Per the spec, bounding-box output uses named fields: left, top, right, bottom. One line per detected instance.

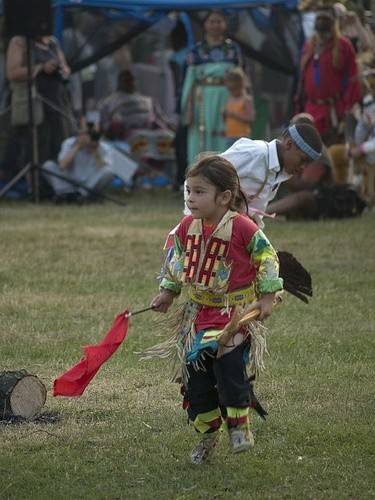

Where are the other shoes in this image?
left=56, top=192, right=106, bottom=206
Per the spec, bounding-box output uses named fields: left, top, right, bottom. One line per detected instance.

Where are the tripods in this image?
left=0, top=36, right=127, bottom=207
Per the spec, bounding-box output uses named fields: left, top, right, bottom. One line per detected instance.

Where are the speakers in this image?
left=3, top=0, right=54, bottom=35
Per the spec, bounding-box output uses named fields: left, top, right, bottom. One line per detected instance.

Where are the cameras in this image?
left=82, top=121, right=101, bottom=141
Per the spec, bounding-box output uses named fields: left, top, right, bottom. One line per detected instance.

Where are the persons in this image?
left=0, top=0, right=374, bottom=219
left=150, top=125, right=323, bottom=465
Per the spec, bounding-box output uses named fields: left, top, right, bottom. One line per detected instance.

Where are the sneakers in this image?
left=192, top=433, right=220, bottom=466
left=231, top=429, right=255, bottom=455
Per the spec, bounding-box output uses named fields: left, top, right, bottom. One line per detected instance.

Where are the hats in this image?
left=313, top=16, right=335, bottom=32
